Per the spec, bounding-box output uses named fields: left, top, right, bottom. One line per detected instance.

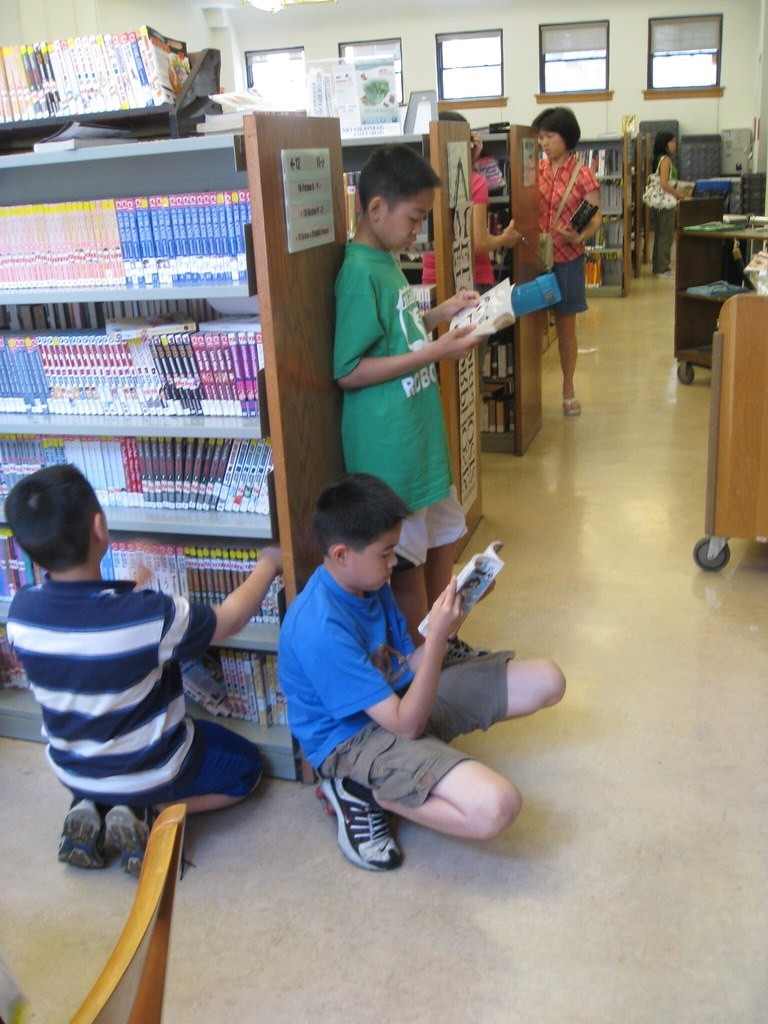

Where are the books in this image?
left=343, top=169, right=436, bottom=342
left=539, top=150, right=635, bottom=288
left=449, top=277, right=515, bottom=338
left=570, top=200, right=599, bottom=234
left=473, top=155, right=516, bottom=433
left=0, top=189, right=287, bottom=730
left=417, top=541, right=504, bottom=639
left=1, top=24, right=307, bottom=155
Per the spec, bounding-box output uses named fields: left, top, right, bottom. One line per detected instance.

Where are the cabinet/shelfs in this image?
left=0, top=48, right=768, bottom=784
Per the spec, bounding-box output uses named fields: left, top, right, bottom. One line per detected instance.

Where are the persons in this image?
left=652, top=131, right=685, bottom=280
left=277, top=473, right=565, bottom=873
left=532, top=106, right=602, bottom=415
left=421, top=111, right=521, bottom=396
left=5, top=463, right=283, bottom=881
left=332, top=144, right=491, bottom=663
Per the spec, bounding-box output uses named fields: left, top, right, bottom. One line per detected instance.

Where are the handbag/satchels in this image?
left=538, top=232, right=554, bottom=273
left=496, top=235, right=562, bottom=318
left=641, top=154, right=679, bottom=210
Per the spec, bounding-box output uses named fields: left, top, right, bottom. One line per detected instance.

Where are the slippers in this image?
left=562, top=397, right=581, bottom=415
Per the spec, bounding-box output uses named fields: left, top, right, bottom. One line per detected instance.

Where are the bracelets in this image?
left=581, top=233, right=585, bottom=242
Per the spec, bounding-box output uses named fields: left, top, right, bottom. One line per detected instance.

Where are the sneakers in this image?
left=441, top=634, right=491, bottom=668
left=314, top=776, right=403, bottom=872
left=56, top=798, right=106, bottom=869
left=103, top=804, right=189, bottom=881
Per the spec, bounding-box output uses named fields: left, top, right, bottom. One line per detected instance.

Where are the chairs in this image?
left=73, top=802, right=188, bottom=1024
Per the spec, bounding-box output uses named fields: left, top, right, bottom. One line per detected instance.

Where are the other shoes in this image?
left=655, top=269, right=675, bottom=280
